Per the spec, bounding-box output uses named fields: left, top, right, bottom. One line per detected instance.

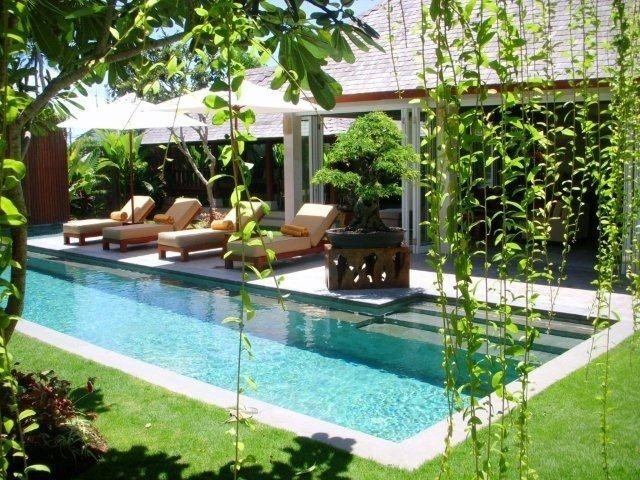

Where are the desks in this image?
left=324, top=244, right=411, bottom=290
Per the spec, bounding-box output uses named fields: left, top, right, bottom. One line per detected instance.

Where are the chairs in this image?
left=522, top=188, right=594, bottom=242
left=225, top=202, right=346, bottom=273
left=63, top=195, right=155, bottom=245
left=158, top=200, right=266, bottom=261
left=103, top=198, right=203, bottom=252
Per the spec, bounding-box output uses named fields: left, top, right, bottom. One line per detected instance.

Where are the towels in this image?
left=211, top=221, right=235, bottom=231
left=281, top=224, right=309, bottom=237
left=153, top=214, right=174, bottom=225
left=110, top=211, right=128, bottom=221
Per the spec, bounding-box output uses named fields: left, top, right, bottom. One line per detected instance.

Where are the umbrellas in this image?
left=56, top=91, right=208, bottom=220
left=156, top=72, right=334, bottom=184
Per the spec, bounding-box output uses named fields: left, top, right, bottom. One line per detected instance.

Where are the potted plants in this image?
left=310, top=111, right=421, bottom=249
